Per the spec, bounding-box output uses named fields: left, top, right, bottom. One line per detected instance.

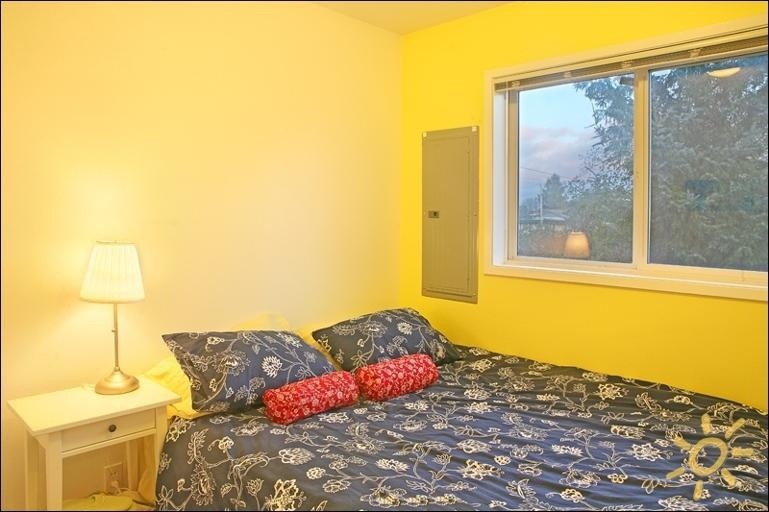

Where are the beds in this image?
left=121, top=324, right=769, bottom=511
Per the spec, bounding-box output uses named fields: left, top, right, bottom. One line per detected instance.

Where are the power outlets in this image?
left=105, top=462, right=123, bottom=494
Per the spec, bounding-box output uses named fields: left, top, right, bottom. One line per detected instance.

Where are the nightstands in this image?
left=6, top=369, right=184, bottom=509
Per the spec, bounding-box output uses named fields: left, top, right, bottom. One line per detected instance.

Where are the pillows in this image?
left=251, top=368, right=362, bottom=429
left=157, top=328, right=338, bottom=417
left=295, top=307, right=392, bottom=374
left=348, top=350, right=442, bottom=401
left=142, top=312, right=337, bottom=422
left=307, top=301, right=464, bottom=377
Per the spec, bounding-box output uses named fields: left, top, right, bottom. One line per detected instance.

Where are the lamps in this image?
left=74, top=236, right=157, bottom=394
